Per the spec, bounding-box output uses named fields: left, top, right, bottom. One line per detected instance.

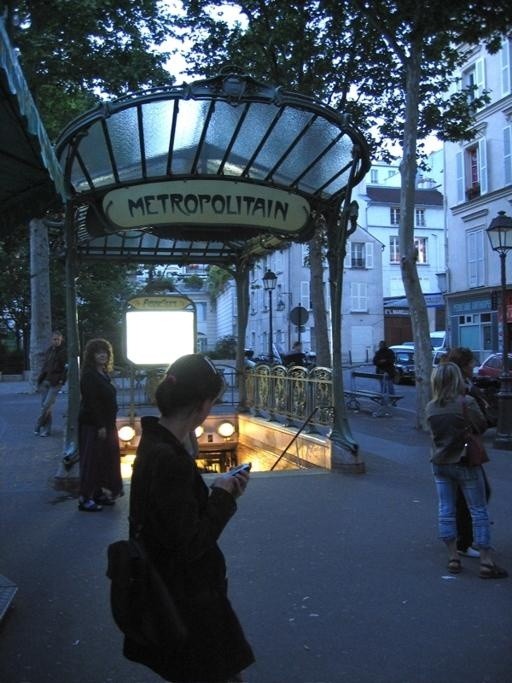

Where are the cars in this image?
left=388, top=328, right=512, bottom=381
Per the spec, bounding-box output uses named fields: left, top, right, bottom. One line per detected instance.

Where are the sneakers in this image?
left=457, top=543, right=481, bottom=559
left=32, top=428, right=48, bottom=437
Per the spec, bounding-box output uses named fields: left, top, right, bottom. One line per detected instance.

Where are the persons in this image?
left=372, top=340, right=397, bottom=406
left=76, top=337, right=126, bottom=512
left=283, top=340, right=306, bottom=367
left=33, top=329, right=68, bottom=438
left=425, top=360, right=508, bottom=580
left=121, top=353, right=257, bottom=682
left=446, top=346, right=492, bottom=559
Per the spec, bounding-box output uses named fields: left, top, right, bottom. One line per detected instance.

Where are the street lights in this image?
left=261, top=268, right=280, bottom=367
left=484, top=209, right=512, bottom=451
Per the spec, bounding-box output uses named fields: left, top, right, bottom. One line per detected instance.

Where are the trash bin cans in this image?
left=471, top=375, right=501, bottom=428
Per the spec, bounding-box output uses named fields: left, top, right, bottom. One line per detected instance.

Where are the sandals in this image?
left=475, top=561, right=506, bottom=580
left=76, top=489, right=115, bottom=511
left=448, top=556, right=464, bottom=574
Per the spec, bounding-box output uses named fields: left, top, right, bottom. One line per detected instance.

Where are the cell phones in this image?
left=222, top=464, right=250, bottom=480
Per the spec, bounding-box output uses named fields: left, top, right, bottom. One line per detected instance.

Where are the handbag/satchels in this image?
left=465, top=429, right=490, bottom=466
left=103, top=540, right=200, bottom=672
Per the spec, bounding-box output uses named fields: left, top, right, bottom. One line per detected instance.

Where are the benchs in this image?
left=342, top=371, right=405, bottom=418
